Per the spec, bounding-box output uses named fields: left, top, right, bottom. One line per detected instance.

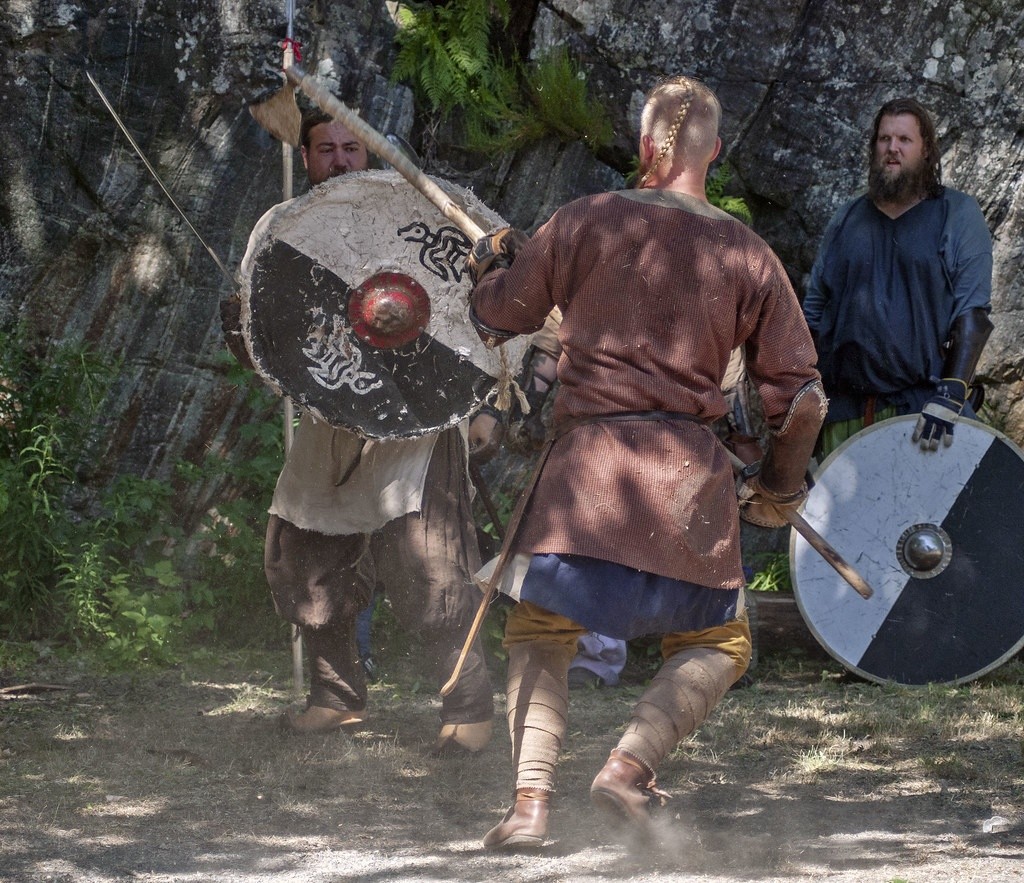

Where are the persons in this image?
left=264, top=82, right=497, bottom=763
left=467, top=75, right=830, bottom=874
left=802, top=97, right=995, bottom=685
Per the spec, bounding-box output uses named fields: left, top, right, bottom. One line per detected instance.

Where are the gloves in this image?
left=508, top=405, right=545, bottom=457
left=468, top=228, right=529, bottom=287
left=912, top=378, right=972, bottom=451
left=219, top=296, right=255, bottom=370
left=737, top=475, right=809, bottom=528
left=468, top=413, right=505, bottom=465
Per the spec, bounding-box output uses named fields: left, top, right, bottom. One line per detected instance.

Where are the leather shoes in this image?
left=590, top=748, right=674, bottom=844
left=483, top=786, right=554, bottom=851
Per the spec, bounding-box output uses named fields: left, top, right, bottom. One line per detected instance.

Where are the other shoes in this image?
left=568, top=668, right=601, bottom=691
left=432, top=719, right=492, bottom=756
left=279, top=705, right=363, bottom=736
left=413, top=671, right=439, bottom=694
